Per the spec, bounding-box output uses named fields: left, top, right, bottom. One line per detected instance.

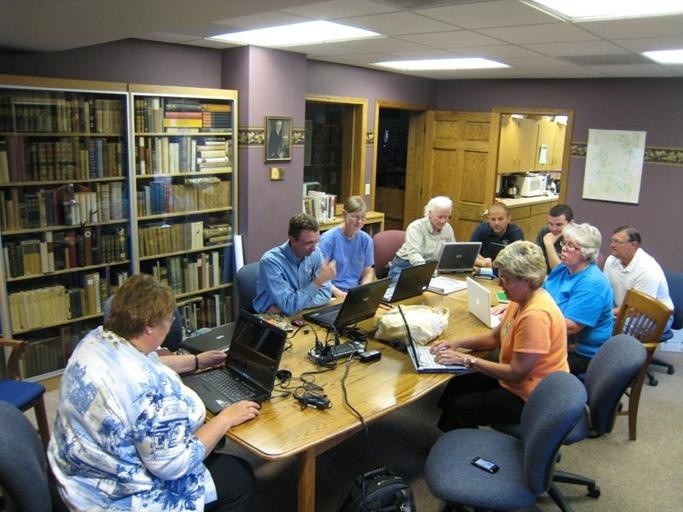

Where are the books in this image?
left=303, top=190, right=338, bottom=223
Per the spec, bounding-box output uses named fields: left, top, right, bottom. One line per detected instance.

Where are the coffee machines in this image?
left=500, top=174, right=519, bottom=199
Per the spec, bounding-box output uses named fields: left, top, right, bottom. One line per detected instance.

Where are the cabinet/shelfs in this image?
left=495, top=115, right=538, bottom=177
left=533, top=119, right=567, bottom=173
left=0, top=72, right=240, bottom=388
left=493, top=198, right=559, bottom=247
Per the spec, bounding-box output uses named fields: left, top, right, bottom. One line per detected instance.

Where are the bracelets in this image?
left=463, top=355, right=476, bottom=368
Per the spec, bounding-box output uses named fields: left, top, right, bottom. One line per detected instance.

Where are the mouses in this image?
left=291, top=319, right=305, bottom=328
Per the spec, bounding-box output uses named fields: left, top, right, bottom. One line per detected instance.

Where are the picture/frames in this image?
left=264, top=114, right=292, bottom=164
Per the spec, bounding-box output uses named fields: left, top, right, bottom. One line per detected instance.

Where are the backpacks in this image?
left=335, top=463, right=417, bottom=511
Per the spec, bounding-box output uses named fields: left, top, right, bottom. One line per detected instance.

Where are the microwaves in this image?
left=514, top=173, right=547, bottom=197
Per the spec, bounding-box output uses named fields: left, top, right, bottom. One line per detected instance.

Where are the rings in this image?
left=443, top=353, right=448, bottom=359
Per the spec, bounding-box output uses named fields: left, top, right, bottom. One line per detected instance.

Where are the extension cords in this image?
left=308, top=339, right=367, bottom=365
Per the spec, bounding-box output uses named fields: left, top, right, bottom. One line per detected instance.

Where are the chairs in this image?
left=487, top=332, right=646, bottom=512
left=643, top=272, right=682, bottom=390
left=373, top=228, right=410, bottom=280
left=0, top=399, right=71, bottom=511
left=0, top=337, right=51, bottom=457
left=610, top=286, right=673, bottom=442
left=422, top=369, right=589, bottom=512
left=102, top=290, right=185, bottom=355
left=234, top=260, right=262, bottom=313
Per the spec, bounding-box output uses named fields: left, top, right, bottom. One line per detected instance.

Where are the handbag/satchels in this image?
left=373, top=303, right=450, bottom=348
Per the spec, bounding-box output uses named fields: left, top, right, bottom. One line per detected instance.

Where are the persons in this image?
left=536, top=205, right=574, bottom=275
left=317, top=196, right=376, bottom=299
left=603, top=226, right=675, bottom=339
left=387, top=196, right=456, bottom=283
left=47, top=274, right=261, bottom=512
left=253, top=213, right=337, bottom=314
left=429, top=240, right=571, bottom=433
left=539, top=222, right=615, bottom=376
left=471, top=202, right=524, bottom=267
left=268, top=119, right=288, bottom=158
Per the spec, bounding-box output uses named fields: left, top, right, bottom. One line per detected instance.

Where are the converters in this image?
left=299, top=392, right=330, bottom=411
left=348, top=332, right=368, bottom=342
left=360, top=349, right=381, bottom=362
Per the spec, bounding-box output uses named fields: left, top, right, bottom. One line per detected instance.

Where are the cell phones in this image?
left=470, top=455, right=500, bottom=474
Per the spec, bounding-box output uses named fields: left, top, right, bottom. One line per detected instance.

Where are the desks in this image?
left=317, top=211, right=386, bottom=239
left=173, top=261, right=525, bottom=511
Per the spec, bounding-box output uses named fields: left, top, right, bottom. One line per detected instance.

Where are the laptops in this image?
left=181, top=322, right=244, bottom=356
left=380, top=260, right=438, bottom=303
left=397, top=305, right=470, bottom=374
left=435, top=242, right=483, bottom=274
left=466, top=276, right=502, bottom=329
left=302, top=275, right=393, bottom=330
left=180, top=307, right=287, bottom=417
